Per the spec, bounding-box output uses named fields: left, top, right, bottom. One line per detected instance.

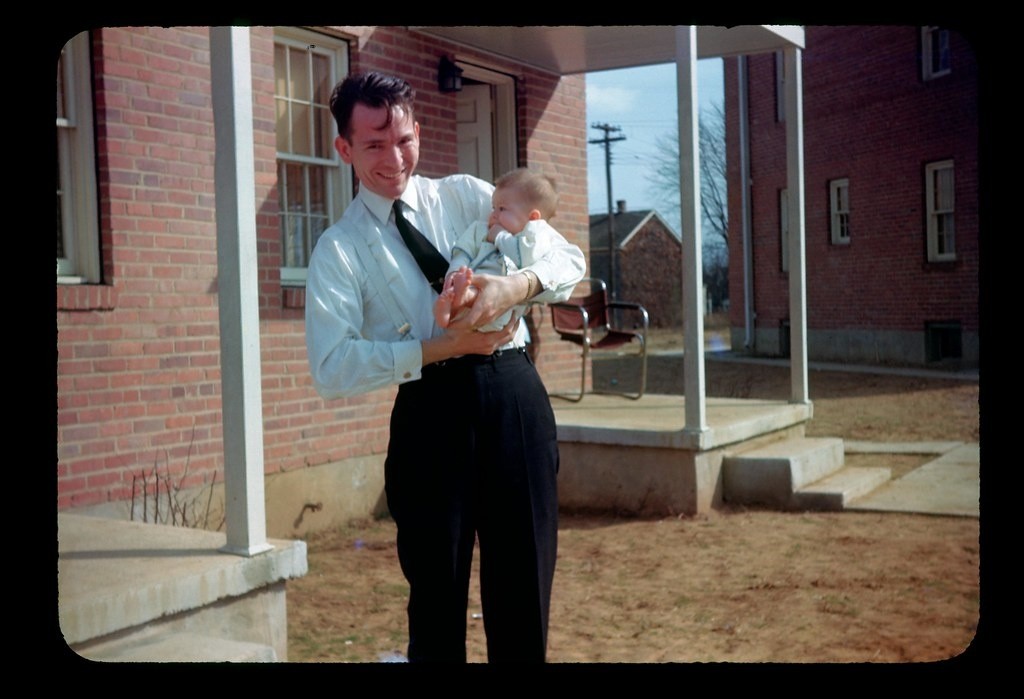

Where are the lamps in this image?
left=438, top=57, right=463, bottom=95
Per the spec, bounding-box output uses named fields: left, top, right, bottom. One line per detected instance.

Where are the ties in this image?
left=391, top=199, right=450, bottom=293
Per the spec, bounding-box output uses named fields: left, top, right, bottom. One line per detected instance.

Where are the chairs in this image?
left=547, top=278, right=649, bottom=403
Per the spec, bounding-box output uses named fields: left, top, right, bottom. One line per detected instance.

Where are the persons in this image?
left=303, top=70, right=587, bottom=663
left=432, top=168, right=559, bottom=331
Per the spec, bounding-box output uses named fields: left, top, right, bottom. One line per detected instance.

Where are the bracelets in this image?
left=522, top=271, right=533, bottom=300
